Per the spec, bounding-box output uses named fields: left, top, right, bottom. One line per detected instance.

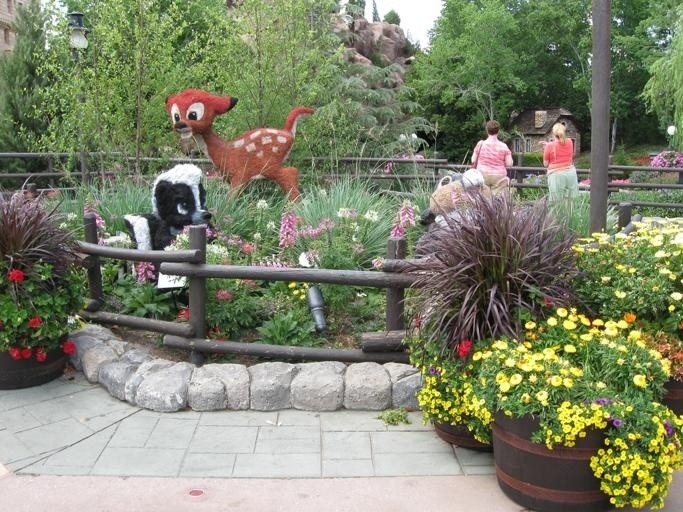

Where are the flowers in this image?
left=654, top=153, right=683, bottom=170
left=402, top=310, right=588, bottom=446
left=621, top=308, right=683, bottom=385
left=471, top=308, right=680, bottom=508
left=0, top=266, right=87, bottom=362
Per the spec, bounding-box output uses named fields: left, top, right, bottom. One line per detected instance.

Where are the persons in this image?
left=538, top=122, right=579, bottom=202
left=471, top=120, right=513, bottom=184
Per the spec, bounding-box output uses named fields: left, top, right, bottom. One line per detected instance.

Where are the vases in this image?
left=1, top=345, right=71, bottom=391
left=485, top=395, right=618, bottom=508
left=652, top=374, right=683, bottom=418
left=658, top=168, right=677, bottom=187
left=422, top=385, right=494, bottom=453
